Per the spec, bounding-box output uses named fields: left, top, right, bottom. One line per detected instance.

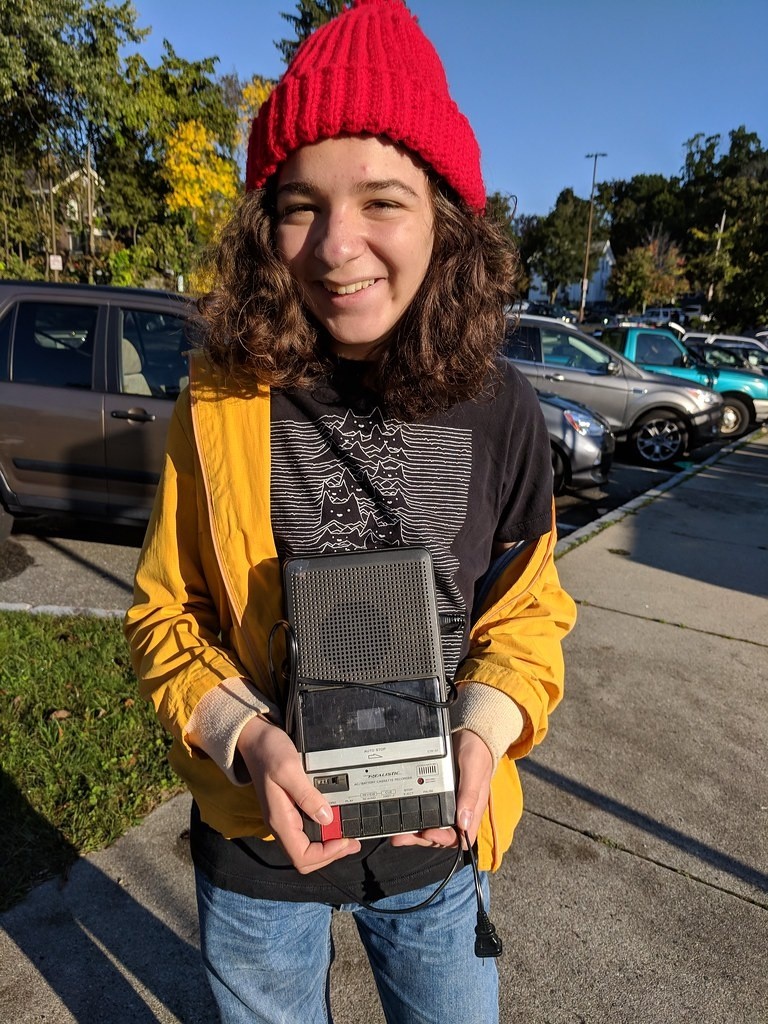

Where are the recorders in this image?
left=284, top=544, right=460, bottom=842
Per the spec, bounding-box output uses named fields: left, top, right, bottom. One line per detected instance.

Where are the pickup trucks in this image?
left=544, top=320, right=768, bottom=441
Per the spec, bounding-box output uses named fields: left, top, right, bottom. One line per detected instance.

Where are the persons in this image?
left=120, top=0, right=580, bottom=1024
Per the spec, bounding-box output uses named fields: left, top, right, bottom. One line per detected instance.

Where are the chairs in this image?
left=121, top=336, right=153, bottom=396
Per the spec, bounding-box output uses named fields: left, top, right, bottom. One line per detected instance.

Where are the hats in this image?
left=244, top=1, right=486, bottom=221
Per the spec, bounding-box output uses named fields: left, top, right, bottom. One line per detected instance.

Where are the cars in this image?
left=501, top=299, right=530, bottom=314
left=534, top=387, right=618, bottom=498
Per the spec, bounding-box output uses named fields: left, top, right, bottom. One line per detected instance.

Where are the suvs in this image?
left=0, top=278, right=207, bottom=540
left=496, top=309, right=727, bottom=470
left=527, top=300, right=579, bottom=326
left=628, top=306, right=690, bottom=329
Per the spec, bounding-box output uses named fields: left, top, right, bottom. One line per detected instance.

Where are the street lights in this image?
left=578, top=152, right=609, bottom=324
